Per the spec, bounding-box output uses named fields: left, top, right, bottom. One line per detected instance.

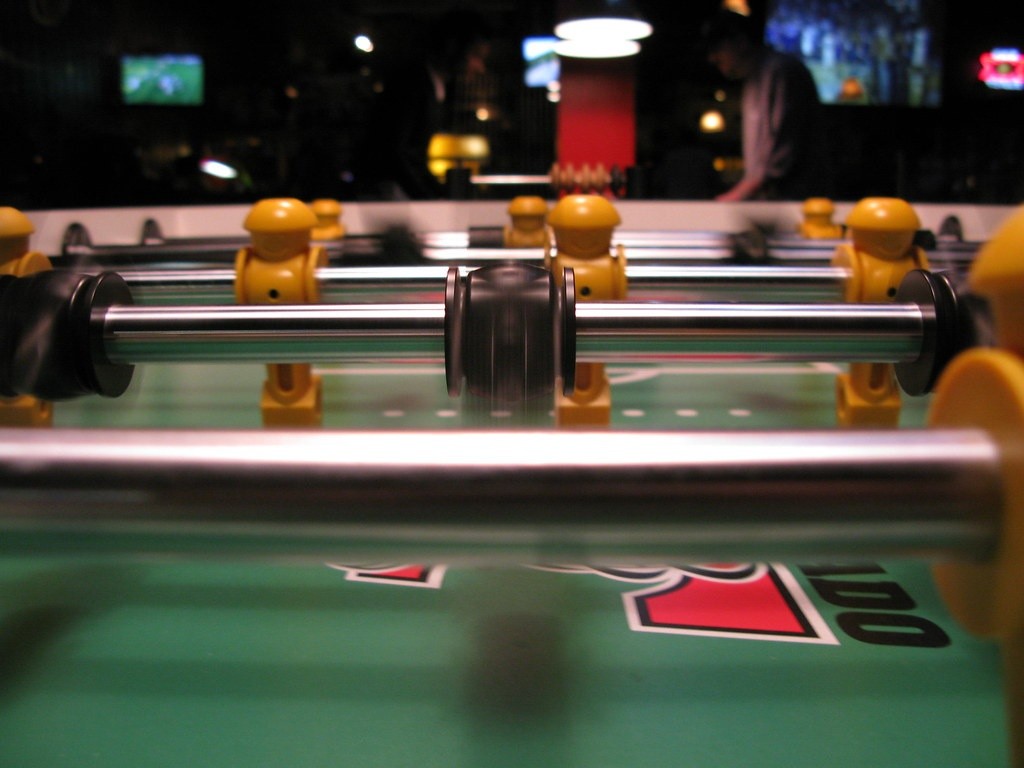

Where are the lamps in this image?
left=551, top=0, right=652, bottom=59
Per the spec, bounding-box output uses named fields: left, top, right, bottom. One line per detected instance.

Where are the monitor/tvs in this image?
left=120, top=55, right=203, bottom=104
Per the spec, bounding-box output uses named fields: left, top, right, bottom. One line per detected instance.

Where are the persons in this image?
left=702, top=11, right=821, bottom=203
left=350, top=9, right=492, bottom=200
